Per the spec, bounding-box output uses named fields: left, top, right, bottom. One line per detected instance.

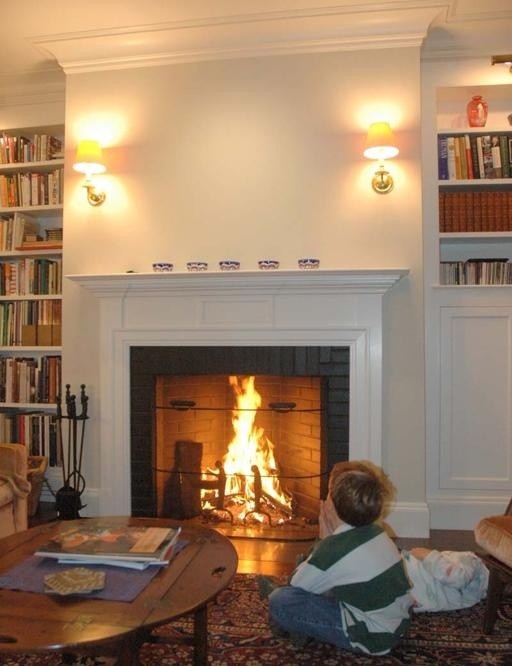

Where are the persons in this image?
left=400, top=547, right=489, bottom=613
left=259, top=461, right=415, bottom=657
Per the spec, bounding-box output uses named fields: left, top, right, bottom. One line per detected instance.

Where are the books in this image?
left=0, top=130, right=64, bottom=467
left=33, top=526, right=182, bottom=570
left=438, top=134, right=512, bottom=285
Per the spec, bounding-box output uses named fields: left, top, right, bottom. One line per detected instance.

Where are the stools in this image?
left=472, top=496, right=510, bottom=635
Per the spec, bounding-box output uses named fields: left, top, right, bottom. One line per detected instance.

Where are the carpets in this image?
left=2, top=575, right=505, bottom=666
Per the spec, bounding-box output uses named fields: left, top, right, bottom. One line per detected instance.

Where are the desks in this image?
left=0, top=518, right=238, bottom=666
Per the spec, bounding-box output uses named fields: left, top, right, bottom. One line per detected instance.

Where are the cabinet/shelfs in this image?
left=421, top=44, right=511, bottom=530
left=0, top=102, right=62, bottom=503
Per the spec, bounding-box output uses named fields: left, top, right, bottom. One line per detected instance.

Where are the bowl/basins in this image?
left=298, top=258, right=320, bottom=269
left=152, top=262, right=173, bottom=272
left=258, top=260, right=279, bottom=269
left=219, top=260, right=240, bottom=271
left=186, top=261, right=208, bottom=272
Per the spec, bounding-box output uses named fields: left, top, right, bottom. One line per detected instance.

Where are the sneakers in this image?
left=255, top=573, right=290, bottom=596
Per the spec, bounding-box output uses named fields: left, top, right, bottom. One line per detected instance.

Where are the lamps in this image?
left=362, top=122, right=401, bottom=195
left=71, top=139, right=108, bottom=207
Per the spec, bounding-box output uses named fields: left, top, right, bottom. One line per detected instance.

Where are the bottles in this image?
left=467, top=95, right=488, bottom=127
